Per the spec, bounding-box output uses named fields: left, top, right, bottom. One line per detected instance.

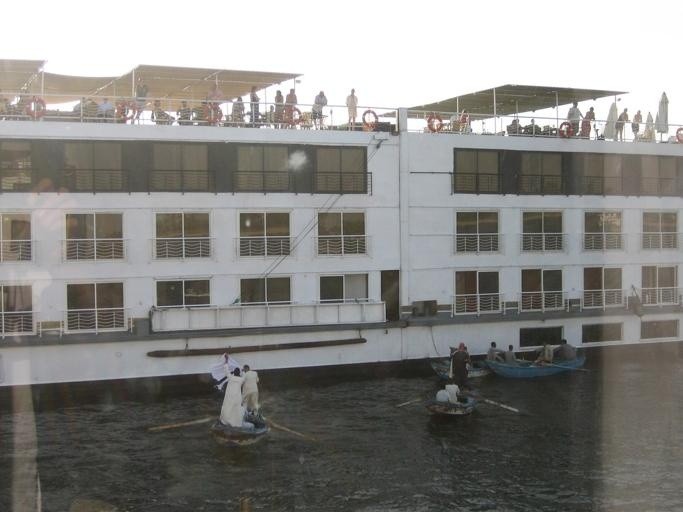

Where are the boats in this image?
left=210, top=412, right=274, bottom=448
left=483, top=352, right=590, bottom=379
left=427, top=358, right=493, bottom=382
left=422, top=391, right=477, bottom=418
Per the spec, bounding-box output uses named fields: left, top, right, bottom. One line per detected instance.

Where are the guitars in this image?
left=630, top=284, right=645, bottom=317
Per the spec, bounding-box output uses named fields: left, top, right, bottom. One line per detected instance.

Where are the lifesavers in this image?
left=558, top=122, right=574, bottom=139
left=427, top=113, right=444, bottom=133
left=117, top=101, right=139, bottom=122
left=676, top=128, right=683, bottom=143
left=285, top=107, right=301, bottom=125
left=202, top=104, right=222, bottom=124
left=25, top=98, right=45, bottom=119
left=362, top=110, right=378, bottom=128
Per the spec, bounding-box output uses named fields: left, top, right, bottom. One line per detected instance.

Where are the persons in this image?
left=435, top=338, right=577, bottom=404
left=567, top=100, right=595, bottom=140
left=74, top=96, right=114, bottom=123
left=614, top=107, right=630, bottom=142
left=511, top=119, right=541, bottom=135
left=312, top=91, right=327, bottom=130
left=0, top=87, right=32, bottom=121
left=218, top=354, right=246, bottom=428
left=632, top=110, right=642, bottom=142
left=151, top=100, right=211, bottom=126
left=239, top=365, right=260, bottom=418
left=266, top=88, right=297, bottom=129
left=346, top=89, right=358, bottom=131
left=232, top=86, right=261, bottom=128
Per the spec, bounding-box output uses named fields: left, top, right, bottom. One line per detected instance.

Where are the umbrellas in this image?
left=656, top=92, right=668, bottom=141
left=646, top=111, right=655, bottom=144
